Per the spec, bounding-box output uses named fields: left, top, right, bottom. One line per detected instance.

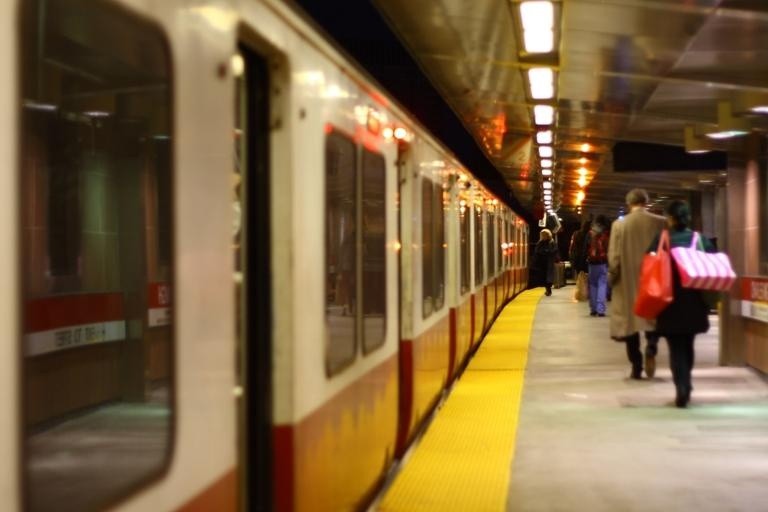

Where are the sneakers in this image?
left=645, top=358, right=655, bottom=378
left=630, top=363, right=643, bottom=379
left=591, top=310, right=605, bottom=317
left=545, top=287, right=552, bottom=296
left=675, top=383, right=693, bottom=408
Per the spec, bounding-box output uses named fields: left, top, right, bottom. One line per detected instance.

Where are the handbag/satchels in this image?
left=671, top=231, right=738, bottom=293
left=574, top=271, right=590, bottom=303
left=633, top=229, right=675, bottom=321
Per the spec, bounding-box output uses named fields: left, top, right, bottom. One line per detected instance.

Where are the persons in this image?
left=534, top=228, right=560, bottom=296
left=364, top=217, right=385, bottom=317
left=644, top=197, right=719, bottom=408
left=555, top=189, right=672, bottom=380
left=342, top=211, right=370, bottom=316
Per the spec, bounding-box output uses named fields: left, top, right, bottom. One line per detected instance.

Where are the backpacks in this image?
left=588, top=230, right=610, bottom=263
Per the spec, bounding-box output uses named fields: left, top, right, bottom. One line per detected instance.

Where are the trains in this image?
left=0, top=1, right=529, bottom=511
